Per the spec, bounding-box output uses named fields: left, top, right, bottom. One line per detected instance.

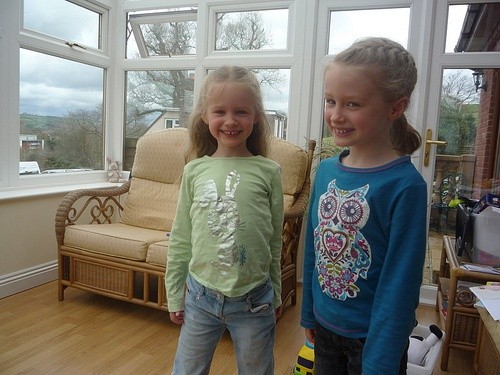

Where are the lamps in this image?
left=472, top=68, right=487, bottom=93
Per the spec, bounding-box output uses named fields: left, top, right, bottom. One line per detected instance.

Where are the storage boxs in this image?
left=459, top=201, right=500, bottom=267
left=406, top=324, right=447, bottom=375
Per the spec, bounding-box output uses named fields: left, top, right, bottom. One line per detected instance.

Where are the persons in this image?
left=164, top=65, right=284, bottom=375
left=302, top=41, right=428, bottom=375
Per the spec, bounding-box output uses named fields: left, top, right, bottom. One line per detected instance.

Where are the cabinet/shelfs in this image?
left=435, top=236, right=500, bottom=375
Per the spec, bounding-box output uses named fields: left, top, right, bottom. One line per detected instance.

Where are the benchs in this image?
left=56, top=126, right=316, bottom=325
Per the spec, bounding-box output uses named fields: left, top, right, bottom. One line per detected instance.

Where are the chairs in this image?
left=431, top=172, right=467, bottom=235
